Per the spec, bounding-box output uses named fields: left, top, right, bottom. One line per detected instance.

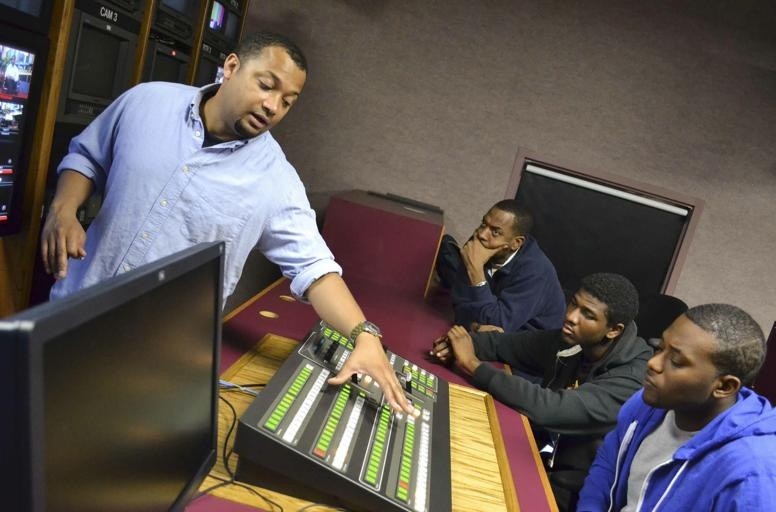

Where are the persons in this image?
left=5, top=56, right=20, bottom=93
left=35, top=32, right=420, bottom=416
left=5, top=110, right=14, bottom=127
left=445, top=200, right=570, bottom=333
left=574, top=303, right=776, bottom=511
left=431, top=271, right=654, bottom=512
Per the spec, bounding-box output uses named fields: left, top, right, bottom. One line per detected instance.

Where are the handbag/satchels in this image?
left=436, top=235, right=466, bottom=289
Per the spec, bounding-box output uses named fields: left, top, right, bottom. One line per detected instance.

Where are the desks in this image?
left=186, top=274, right=561, bottom=512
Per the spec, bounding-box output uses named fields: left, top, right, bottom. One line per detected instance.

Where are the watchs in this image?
left=348, top=319, right=383, bottom=348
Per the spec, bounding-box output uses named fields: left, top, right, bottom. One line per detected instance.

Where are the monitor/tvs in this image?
left=68, top=13, right=139, bottom=115
left=94, top=0, right=145, bottom=20
left=156, top=0, right=197, bottom=40
left=1, top=28, right=51, bottom=234
left=146, top=43, right=191, bottom=84
left=0, top=239, right=227, bottom=512
left=0, top=0, right=54, bottom=34
left=209, top=1, right=240, bottom=41
left=208, top=66, right=224, bottom=84
